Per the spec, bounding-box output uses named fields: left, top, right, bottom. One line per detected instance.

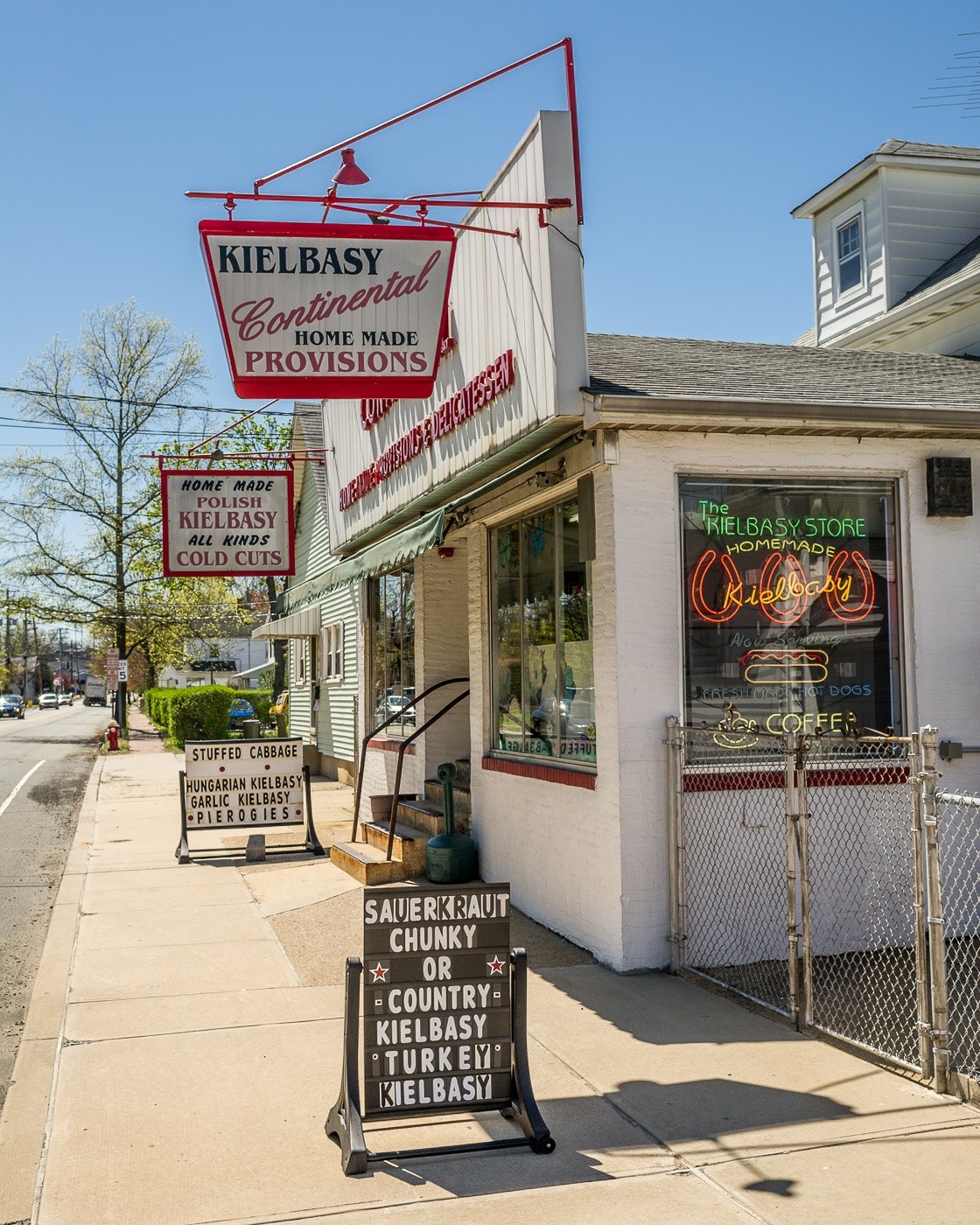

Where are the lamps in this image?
left=211, top=441, right=223, bottom=459
left=367, top=214, right=390, bottom=225
left=331, top=147, right=370, bottom=196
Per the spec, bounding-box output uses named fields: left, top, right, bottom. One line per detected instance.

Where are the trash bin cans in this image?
left=370, top=793, right=419, bottom=824
left=242, top=719, right=262, bottom=738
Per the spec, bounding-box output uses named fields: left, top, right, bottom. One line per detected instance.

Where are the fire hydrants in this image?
left=106, top=720, right=121, bottom=752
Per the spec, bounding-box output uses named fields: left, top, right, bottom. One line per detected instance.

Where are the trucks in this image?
left=83, top=677, right=107, bottom=706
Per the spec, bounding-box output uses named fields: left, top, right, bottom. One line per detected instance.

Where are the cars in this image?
left=378, top=695, right=416, bottom=723
left=38, top=693, right=59, bottom=710
left=532, top=698, right=595, bottom=740
left=58, top=694, right=73, bottom=706
left=0, top=694, right=26, bottom=719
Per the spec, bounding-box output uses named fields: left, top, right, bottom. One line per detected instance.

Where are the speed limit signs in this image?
left=118, top=660, right=128, bottom=681
